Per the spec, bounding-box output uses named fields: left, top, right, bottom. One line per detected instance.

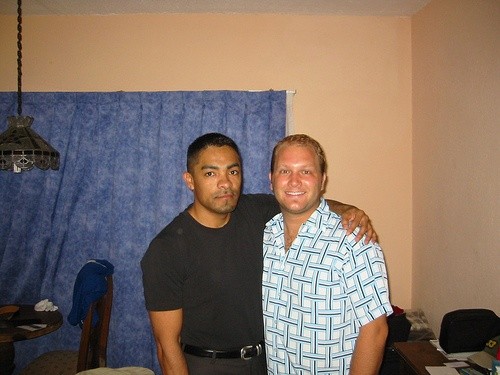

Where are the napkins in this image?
left=34, top=298, right=58, bottom=312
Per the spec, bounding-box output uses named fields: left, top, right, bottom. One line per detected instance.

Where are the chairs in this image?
left=23, top=259, right=112, bottom=375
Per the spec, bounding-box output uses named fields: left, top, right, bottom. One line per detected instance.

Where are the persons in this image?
left=144, top=131, right=379, bottom=375
left=262, top=134, right=394, bottom=374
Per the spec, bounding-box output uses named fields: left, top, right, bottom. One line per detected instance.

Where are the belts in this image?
left=180, top=340, right=265, bottom=360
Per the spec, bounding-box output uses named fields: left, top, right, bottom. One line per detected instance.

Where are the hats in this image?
left=467, top=336, right=500, bottom=370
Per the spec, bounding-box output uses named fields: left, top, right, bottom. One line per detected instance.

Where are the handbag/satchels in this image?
left=439, top=309, right=500, bottom=354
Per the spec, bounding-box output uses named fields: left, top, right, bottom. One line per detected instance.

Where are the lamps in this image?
left=0, top=0, right=61, bottom=174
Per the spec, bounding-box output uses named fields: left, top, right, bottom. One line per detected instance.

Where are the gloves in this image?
left=34, top=299, right=58, bottom=312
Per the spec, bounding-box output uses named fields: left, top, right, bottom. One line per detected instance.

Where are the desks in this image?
left=391, top=341, right=469, bottom=375
left=0, top=304, right=64, bottom=375
